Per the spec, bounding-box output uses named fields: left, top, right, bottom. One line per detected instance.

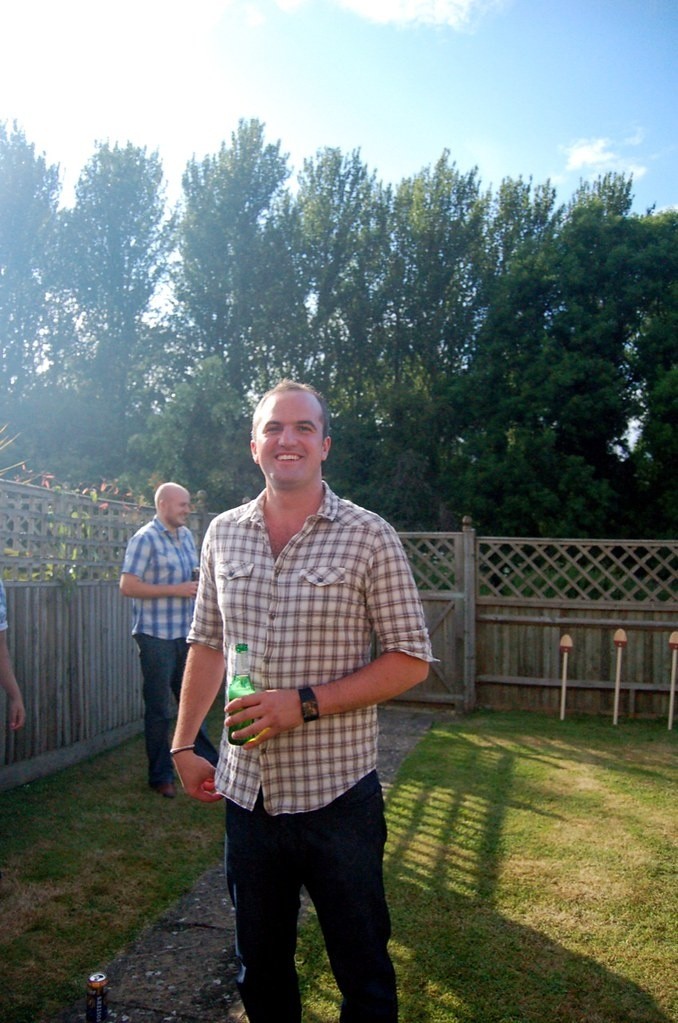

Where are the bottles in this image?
left=228, top=643, right=257, bottom=746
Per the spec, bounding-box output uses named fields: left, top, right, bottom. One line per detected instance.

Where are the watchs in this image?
left=298, top=687, right=320, bottom=723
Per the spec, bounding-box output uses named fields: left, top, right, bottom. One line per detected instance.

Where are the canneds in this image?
left=85, top=972, right=108, bottom=1023
left=192, top=567, right=200, bottom=582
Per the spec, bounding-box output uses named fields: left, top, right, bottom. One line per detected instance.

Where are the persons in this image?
left=120, top=482, right=220, bottom=798
left=171, top=380, right=439, bottom=1023
left=0, top=578, right=27, bottom=733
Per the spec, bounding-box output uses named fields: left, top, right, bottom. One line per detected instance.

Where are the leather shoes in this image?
left=156, top=782, right=176, bottom=799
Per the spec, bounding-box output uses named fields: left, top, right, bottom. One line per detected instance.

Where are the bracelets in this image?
left=170, top=744, right=195, bottom=754
